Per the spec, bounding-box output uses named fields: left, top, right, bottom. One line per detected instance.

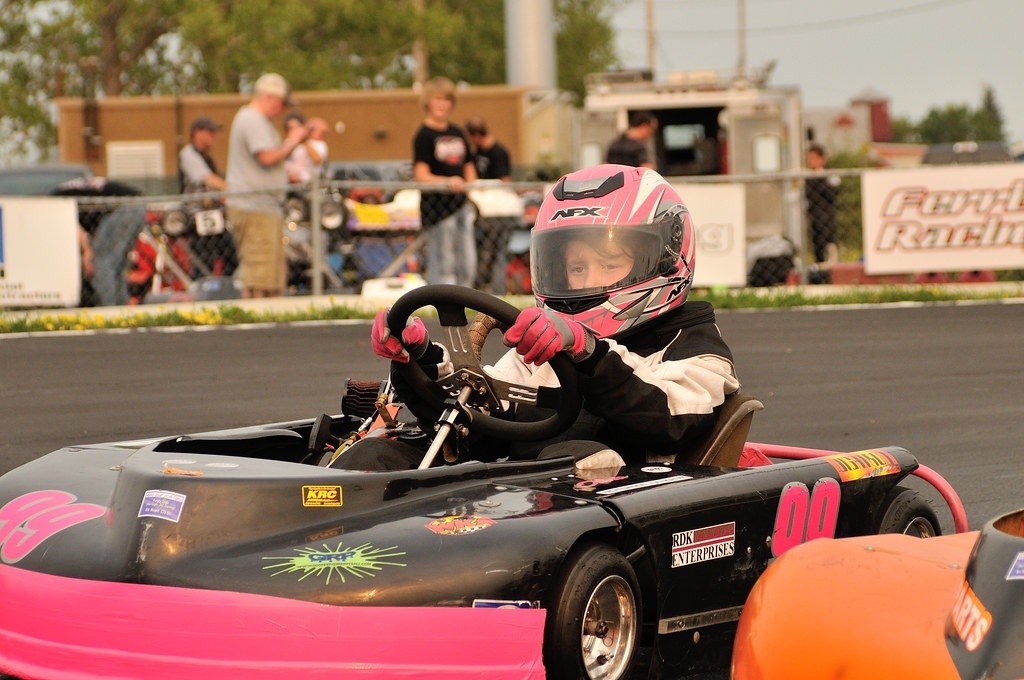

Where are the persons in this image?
left=331, top=164, right=740, bottom=470
left=598, top=117, right=658, bottom=167
left=413, top=78, right=513, bottom=288
left=49, top=177, right=151, bottom=309
left=798, top=146, right=840, bottom=283
left=180, top=73, right=328, bottom=299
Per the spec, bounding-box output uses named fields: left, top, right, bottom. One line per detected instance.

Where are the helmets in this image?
left=530, top=164, right=697, bottom=339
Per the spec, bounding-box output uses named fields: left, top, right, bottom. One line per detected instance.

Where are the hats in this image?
left=254, top=72, right=293, bottom=105
left=191, top=115, right=222, bottom=132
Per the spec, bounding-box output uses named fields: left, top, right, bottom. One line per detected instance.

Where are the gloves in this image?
left=371, top=306, right=431, bottom=362
left=504, top=305, right=597, bottom=366
